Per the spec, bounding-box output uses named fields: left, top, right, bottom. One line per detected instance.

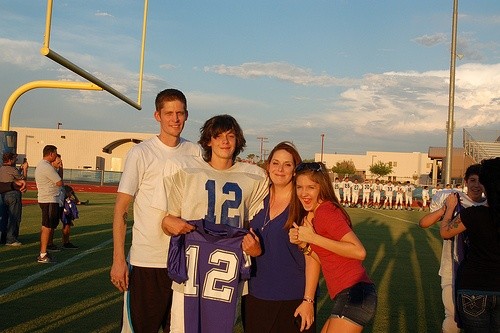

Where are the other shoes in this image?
left=6, top=241, right=23, bottom=246
left=47, top=244, right=63, bottom=251
left=64, top=243, right=80, bottom=249
left=37, top=253, right=58, bottom=264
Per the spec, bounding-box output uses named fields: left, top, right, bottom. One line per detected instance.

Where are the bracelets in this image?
left=299, top=244, right=313, bottom=256
left=303, top=298, right=314, bottom=304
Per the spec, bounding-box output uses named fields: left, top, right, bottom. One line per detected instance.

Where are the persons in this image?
left=289, top=162, right=377, bottom=333
left=440, top=157, right=500, bottom=333
left=443, top=184, right=451, bottom=189
left=111, top=89, right=204, bottom=333
left=332, top=177, right=415, bottom=212
left=0, top=152, right=27, bottom=246
left=456, top=184, right=460, bottom=189
left=161, top=115, right=270, bottom=333
left=20, top=157, right=29, bottom=181
left=242, top=141, right=321, bottom=333
left=76, top=200, right=89, bottom=206
left=419, top=163, right=488, bottom=333
left=35, top=145, right=79, bottom=264
left=436, top=184, right=440, bottom=189
left=430, top=166, right=442, bottom=186
left=422, top=186, right=429, bottom=212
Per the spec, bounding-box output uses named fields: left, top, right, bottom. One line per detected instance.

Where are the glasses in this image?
left=294, top=162, right=324, bottom=174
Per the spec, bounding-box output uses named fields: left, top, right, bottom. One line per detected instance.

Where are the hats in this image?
left=3, top=152, right=18, bottom=161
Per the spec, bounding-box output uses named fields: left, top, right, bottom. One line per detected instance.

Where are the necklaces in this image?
left=261, top=202, right=284, bottom=232
